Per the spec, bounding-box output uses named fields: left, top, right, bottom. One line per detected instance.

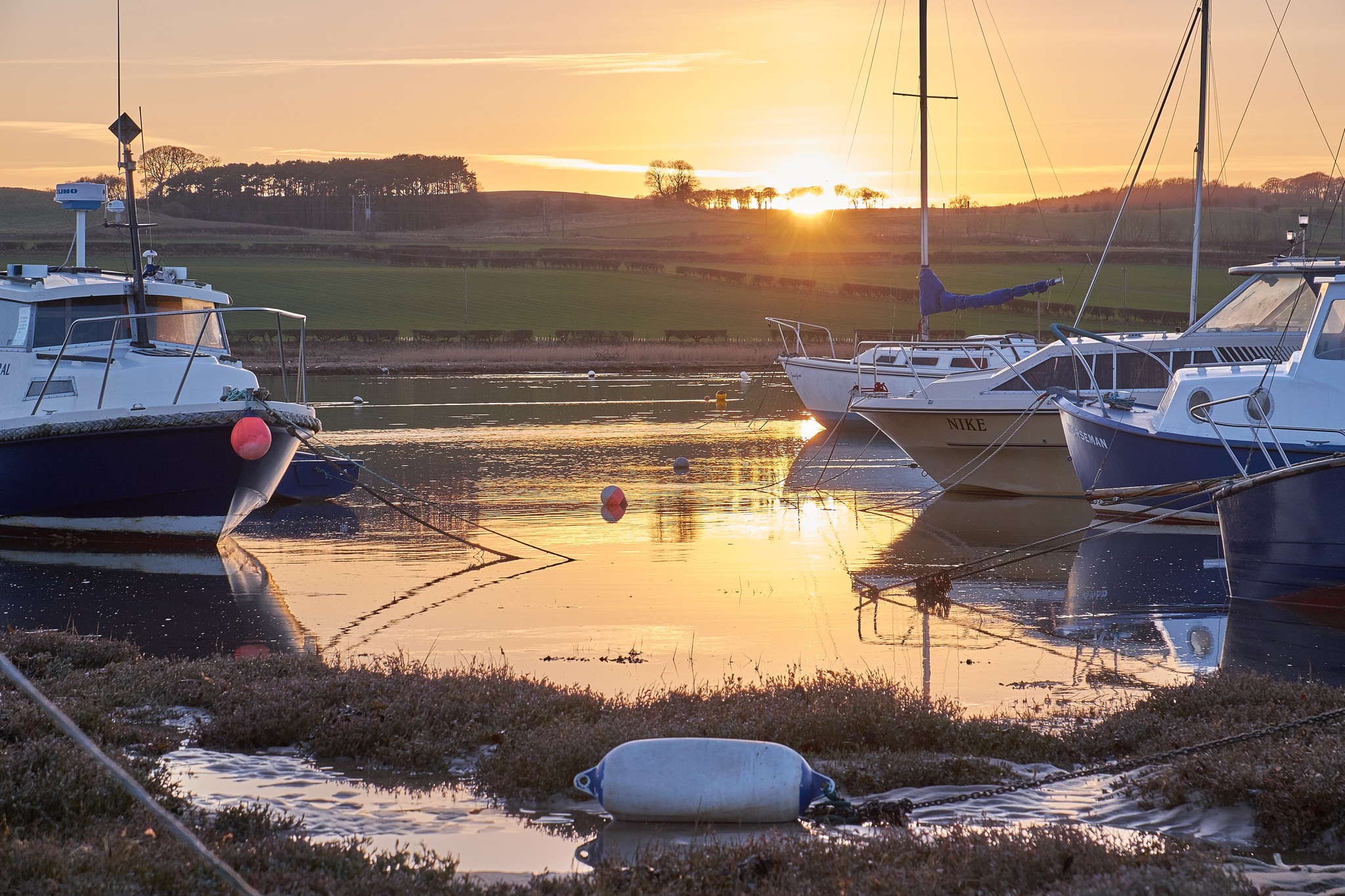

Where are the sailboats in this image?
left=785, top=429, right=1345, bottom=734
left=762, top=0, right=1345, bottom=607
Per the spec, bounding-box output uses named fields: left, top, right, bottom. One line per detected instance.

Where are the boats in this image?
left=1, top=0, right=364, bottom=549
left=1, top=535, right=333, bottom=663
